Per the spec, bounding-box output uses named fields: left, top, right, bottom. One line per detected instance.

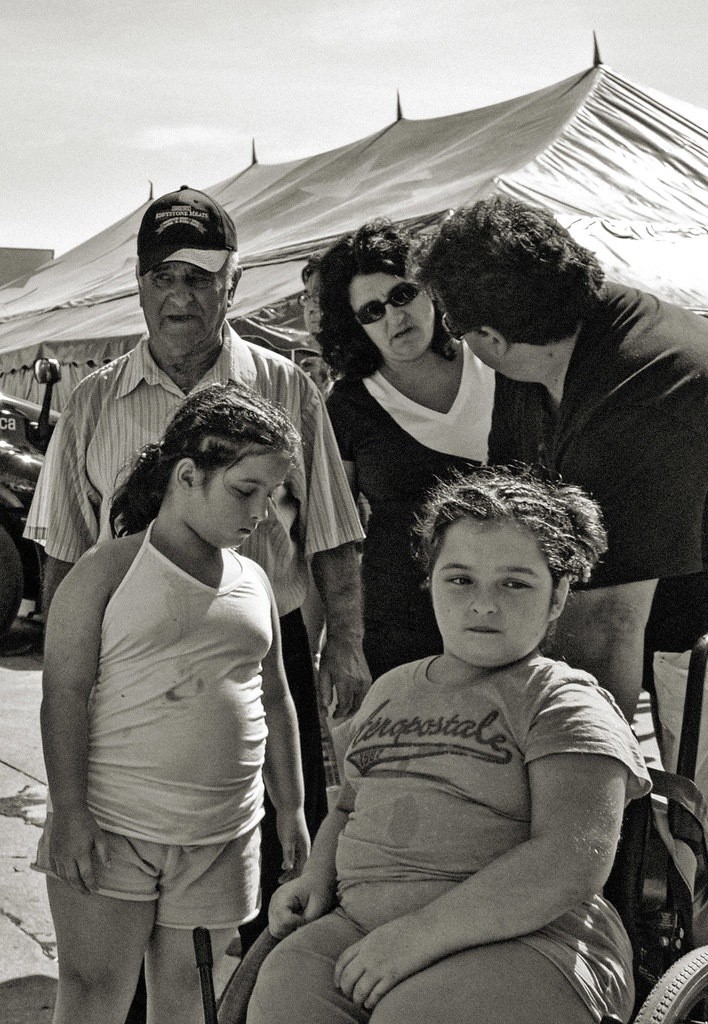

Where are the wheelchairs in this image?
left=192, top=761, right=708, bottom=1024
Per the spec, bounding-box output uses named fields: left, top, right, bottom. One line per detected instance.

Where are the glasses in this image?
left=442, top=312, right=480, bottom=341
left=354, top=282, right=420, bottom=324
left=298, top=293, right=319, bottom=307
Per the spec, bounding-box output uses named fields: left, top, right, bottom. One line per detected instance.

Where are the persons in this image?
left=31, top=182, right=706, bottom=1024
left=242, top=469, right=654, bottom=1024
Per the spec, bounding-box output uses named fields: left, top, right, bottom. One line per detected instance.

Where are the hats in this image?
left=137, top=185, right=238, bottom=277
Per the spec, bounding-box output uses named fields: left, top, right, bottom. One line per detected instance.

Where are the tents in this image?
left=0, top=31, right=707, bottom=417
